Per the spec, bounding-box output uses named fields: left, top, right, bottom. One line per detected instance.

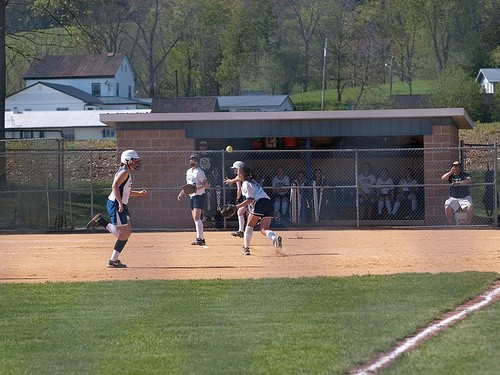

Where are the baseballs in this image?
left=226, top=145, right=233, bottom=152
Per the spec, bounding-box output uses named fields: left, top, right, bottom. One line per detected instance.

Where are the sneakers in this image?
left=273, top=236, right=282, bottom=254
left=243, top=247, right=250, bottom=255
left=231, top=230, right=244, bottom=238
left=85, top=212, right=103, bottom=230
left=108, top=259, right=126, bottom=268
left=191, top=239, right=205, bottom=244
left=196, top=238, right=202, bottom=245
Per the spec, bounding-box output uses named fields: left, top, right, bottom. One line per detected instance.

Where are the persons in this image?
left=271, top=167, right=322, bottom=226
left=219, top=164, right=283, bottom=255
left=376, top=168, right=394, bottom=215
left=86, top=150, right=147, bottom=268
left=178, top=155, right=207, bottom=246
left=357, top=162, right=377, bottom=218
left=230, top=161, right=248, bottom=238
left=390, top=168, right=419, bottom=216
left=441, top=161, right=474, bottom=225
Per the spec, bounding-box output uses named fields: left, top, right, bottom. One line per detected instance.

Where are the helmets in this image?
left=230, top=161, right=244, bottom=169
left=120, top=149, right=140, bottom=165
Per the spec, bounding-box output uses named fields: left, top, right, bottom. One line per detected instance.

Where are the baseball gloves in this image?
left=219, top=204, right=236, bottom=218
left=183, top=184, right=197, bottom=194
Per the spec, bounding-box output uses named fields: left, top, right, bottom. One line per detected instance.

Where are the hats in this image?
left=188, top=153, right=201, bottom=161
